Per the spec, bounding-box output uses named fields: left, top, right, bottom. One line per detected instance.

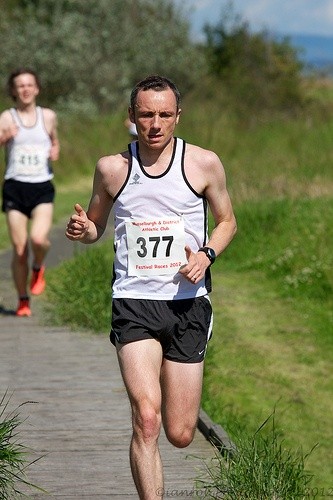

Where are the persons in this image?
left=65, top=76, right=238, bottom=500
left=0, top=67, right=61, bottom=317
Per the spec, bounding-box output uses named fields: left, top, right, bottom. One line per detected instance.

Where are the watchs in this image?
left=198, top=247, right=216, bottom=266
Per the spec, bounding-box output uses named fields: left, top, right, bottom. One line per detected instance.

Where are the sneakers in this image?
left=16, top=297, right=31, bottom=316
left=30, top=265, right=45, bottom=295
